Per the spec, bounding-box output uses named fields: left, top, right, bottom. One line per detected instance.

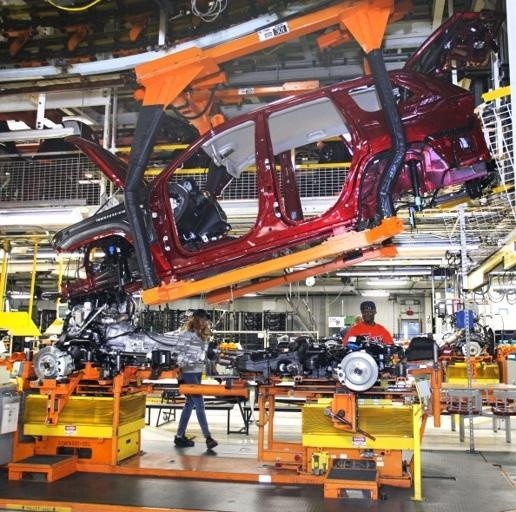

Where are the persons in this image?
left=173, top=308, right=219, bottom=448
left=340, top=300, right=395, bottom=348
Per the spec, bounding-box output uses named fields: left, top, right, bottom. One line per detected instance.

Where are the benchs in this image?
left=273, top=397, right=319, bottom=402
left=144, top=404, right=234, bottom=435
left=243, top=404, right=302, bottom=435
left=163, top=396, right=219, bottom=422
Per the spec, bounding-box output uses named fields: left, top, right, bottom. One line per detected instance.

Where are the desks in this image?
left=152, top=387, right=253, bottom=432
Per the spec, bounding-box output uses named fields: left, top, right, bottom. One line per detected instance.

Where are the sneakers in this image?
left=207, top=440, right=218, bottom=448
left=175, top=434, right=192, bottom=447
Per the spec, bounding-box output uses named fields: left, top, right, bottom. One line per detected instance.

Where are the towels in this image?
left=416, top=380, right=431, bottom=398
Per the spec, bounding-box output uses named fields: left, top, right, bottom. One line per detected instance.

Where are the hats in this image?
left=193, top=308, right=210, bottom=321
